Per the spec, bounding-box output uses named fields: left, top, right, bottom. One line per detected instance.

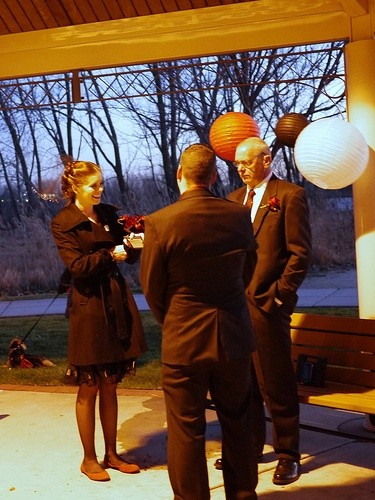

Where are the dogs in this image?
left=2, top=338, right=55, bottom=367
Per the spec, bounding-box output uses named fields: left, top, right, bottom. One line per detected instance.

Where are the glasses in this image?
left=232, top=152, right=267, bottom=168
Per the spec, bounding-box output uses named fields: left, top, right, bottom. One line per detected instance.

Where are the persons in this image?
left=214, top=137, right=313, bottom=485
left=51, top=161, right=148, bottom=481
left=139, top=143, right=267, bottom=500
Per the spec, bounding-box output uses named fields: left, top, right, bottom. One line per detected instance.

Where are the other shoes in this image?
left=80, top=462, right=111, bottom=481
left=103, top=454, right=140, bottom=473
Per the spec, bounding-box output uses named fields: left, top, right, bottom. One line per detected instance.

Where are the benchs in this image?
left=205, top=313, right=375, bottom=443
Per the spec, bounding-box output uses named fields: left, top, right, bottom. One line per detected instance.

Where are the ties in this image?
left=244, top=189, right=256, bottom=210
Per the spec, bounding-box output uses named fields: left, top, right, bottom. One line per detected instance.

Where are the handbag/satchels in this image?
left=296, top=354, right=328, bottom=387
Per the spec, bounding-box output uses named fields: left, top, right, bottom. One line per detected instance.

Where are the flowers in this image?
left=118, top=214, right=145, bottom=232
left=259, top=195, right=280, bottom=211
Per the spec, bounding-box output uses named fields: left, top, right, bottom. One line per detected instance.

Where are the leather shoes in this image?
left=273, top=459, right=302, bottom=485
left=214, top=454, right=264, bottom=467
